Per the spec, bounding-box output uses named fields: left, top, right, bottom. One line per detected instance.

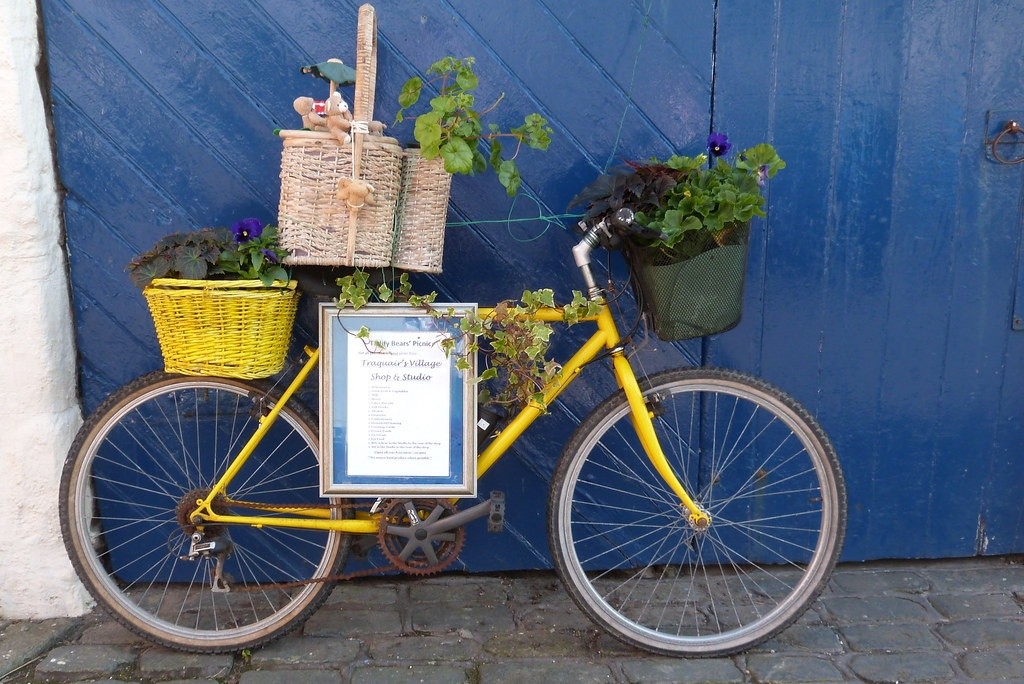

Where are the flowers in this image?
left=130, top=216, right=298, bottom=290
left=565, top=131, right=786, bottom=271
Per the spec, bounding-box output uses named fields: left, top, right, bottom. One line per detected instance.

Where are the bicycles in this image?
left=58, top=204, right=849, bottom=659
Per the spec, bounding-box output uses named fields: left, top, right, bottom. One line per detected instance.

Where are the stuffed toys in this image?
left=335, top=176, right=374, bottom=212
left=292, top=91, right=353, bottom=143
left=367, top=120, right=387, bottom=137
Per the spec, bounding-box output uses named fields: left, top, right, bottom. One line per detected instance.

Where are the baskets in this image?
left=622, top=217, right=752, bottom=340
left=275, top=3, right=402, bottom=268
left=392, top=147, right=455, bottom=273
left=142, top=277, right=301, bottom=380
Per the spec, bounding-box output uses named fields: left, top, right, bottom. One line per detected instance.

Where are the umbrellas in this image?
left=301, top=58, right=357, bottom=98
